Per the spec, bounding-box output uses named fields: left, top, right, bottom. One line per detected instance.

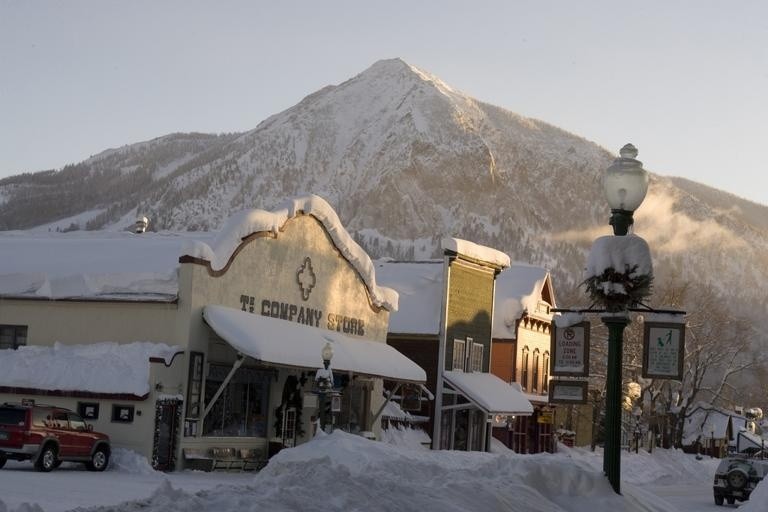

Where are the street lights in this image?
left=321, top=342, right=333, bottom=434
left=602, top=143, right=649, bottom=494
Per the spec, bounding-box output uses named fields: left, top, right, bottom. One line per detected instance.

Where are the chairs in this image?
left=185, top=446, right=270, bottom=473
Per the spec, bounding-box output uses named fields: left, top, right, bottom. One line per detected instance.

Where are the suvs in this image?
left=0, top=399, right=112, bottom=472
left=713, top=457, right=768, bottom=505
